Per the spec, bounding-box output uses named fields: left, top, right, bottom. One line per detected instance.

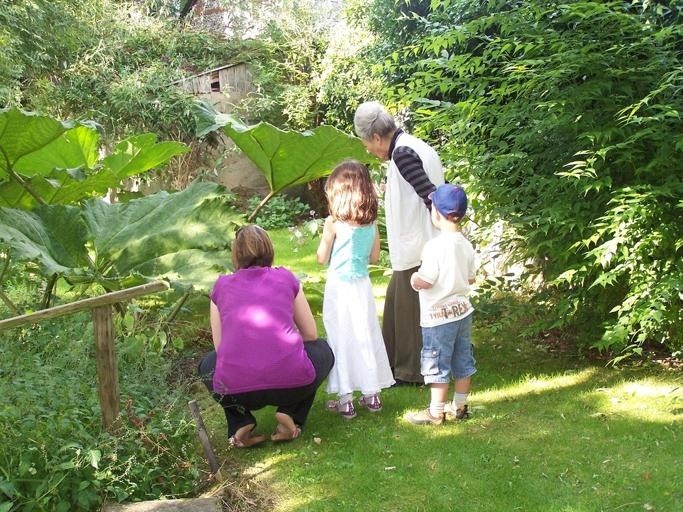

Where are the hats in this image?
left=429, top=185, right=468, bottom=222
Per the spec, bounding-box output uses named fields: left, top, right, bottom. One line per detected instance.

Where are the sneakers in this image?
left=406, top=408, right=444, bottom=425
left=443, top=401, right=468, bottom=421
left=326, top=398, right=359, bottom=420
left=356, top=395, right=383, bottom=412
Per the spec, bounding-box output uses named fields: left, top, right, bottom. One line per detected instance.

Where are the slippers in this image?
left=228, top=433, right=266, bottom=448
left=270, top=424, right=301, bottom=443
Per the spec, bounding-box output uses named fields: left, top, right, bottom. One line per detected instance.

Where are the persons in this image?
left=196, top=225, right=335, bottom=446
left=352, top=100, right=444, bottom=390
left=403, top=182, right=483, bottom=426
left=313, top=161, right=399, bottom=419
